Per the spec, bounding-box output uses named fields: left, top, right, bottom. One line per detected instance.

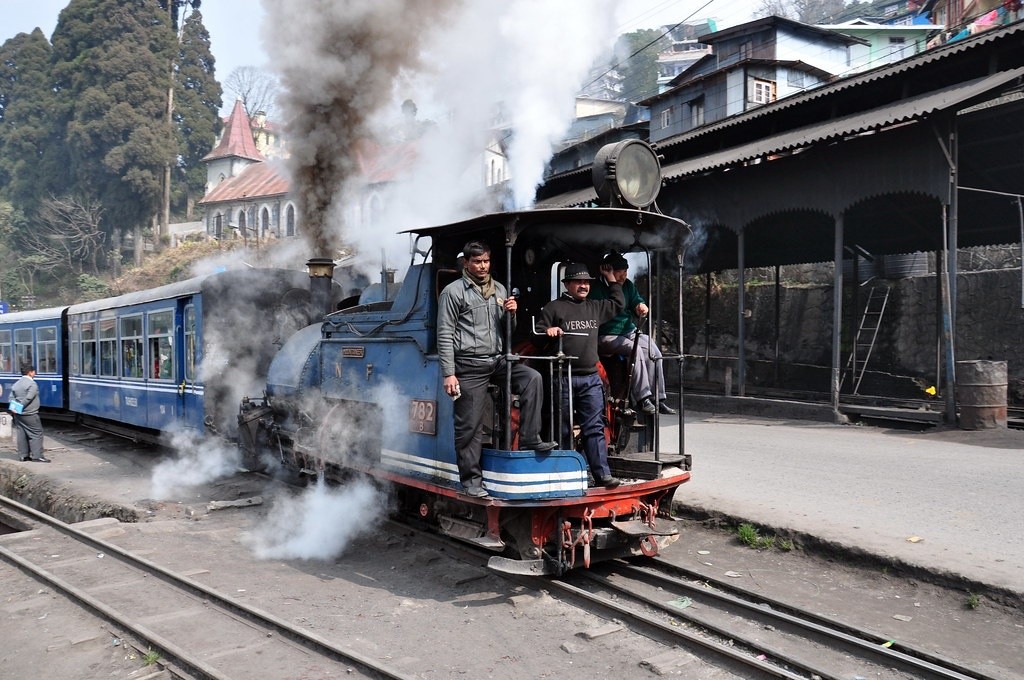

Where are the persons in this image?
left=598, top=254, right=678, bottom=416
left=537, top=261, right=625, bottom=487
left=437, top=242, right=560, bottom=498
left=9, top=365, right=51, bottom=463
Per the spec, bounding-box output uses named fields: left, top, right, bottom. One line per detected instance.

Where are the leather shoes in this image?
left=639, top=398, right=658, bottom=415
left=19, top=456, right=32, bottom=462
left=32, top=457, right=51, bottom=463
left=654, top=402, right=677, bottom=415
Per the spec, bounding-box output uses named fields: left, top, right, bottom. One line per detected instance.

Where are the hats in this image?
left=562, top=263, right=596, bottom=282
left=605, top=249, right=630, bottom=270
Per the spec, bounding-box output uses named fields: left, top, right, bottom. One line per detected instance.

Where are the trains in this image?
left=0, top=137, right=694, bottom=579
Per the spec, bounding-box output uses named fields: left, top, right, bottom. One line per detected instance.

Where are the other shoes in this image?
left=462, top=487, right=493, bottom=498
left=596, top=474, right=625, bottom=487
left=518, top=441, right=559, bottom=453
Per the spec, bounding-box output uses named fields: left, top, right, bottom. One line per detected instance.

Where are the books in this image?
left=9, top=400, right=23, bottom=414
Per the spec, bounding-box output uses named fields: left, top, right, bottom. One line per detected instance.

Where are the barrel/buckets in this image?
left=956, top=360, right=1008, bottom=431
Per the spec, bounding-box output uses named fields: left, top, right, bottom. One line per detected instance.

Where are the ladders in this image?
left=839, top=287, right=893, bottom=395
